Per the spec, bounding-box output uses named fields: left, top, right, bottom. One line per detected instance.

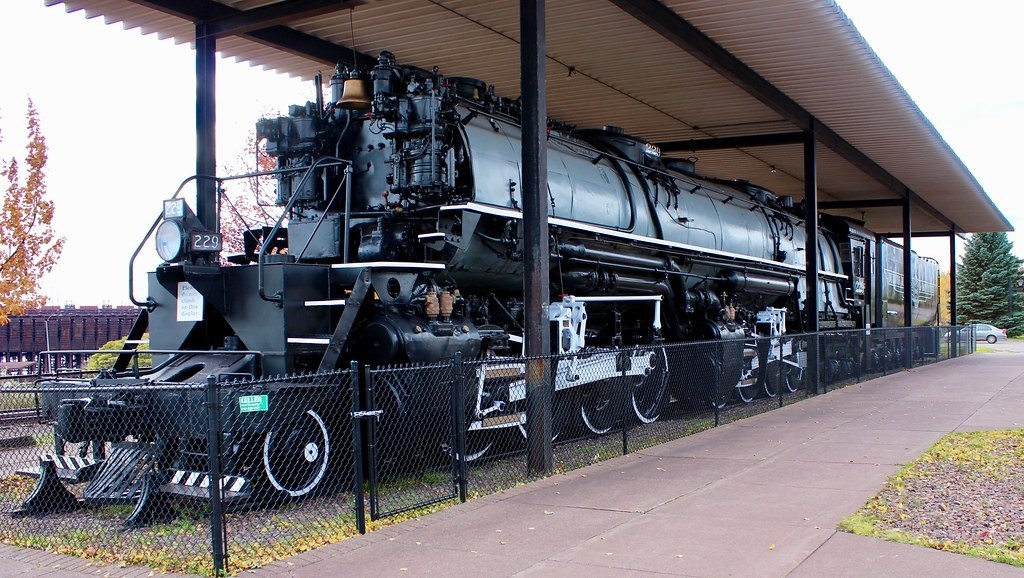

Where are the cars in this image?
left=944, top=324, right=1006, bottom=344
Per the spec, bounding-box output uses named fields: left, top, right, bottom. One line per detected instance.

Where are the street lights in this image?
left=44, top=314, right=57, bottom=374
left=1009, top=276, right=1024, bottom=328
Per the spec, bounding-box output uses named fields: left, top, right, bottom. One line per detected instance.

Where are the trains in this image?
left=32, top=51, right=941, bottom=511
